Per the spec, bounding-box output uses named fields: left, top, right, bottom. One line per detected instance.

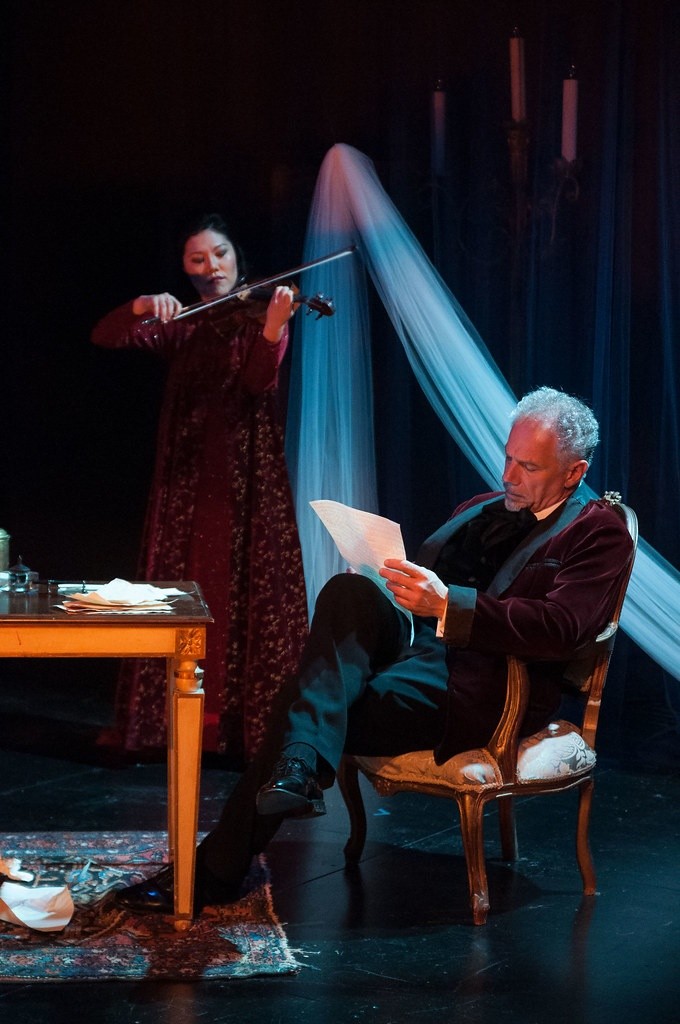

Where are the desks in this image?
left=0, top=577, right=214, bottom=930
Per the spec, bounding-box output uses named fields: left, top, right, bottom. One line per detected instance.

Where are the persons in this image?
left=116, top=388, right=634, bottom=914
left=85, top=218, right=310, bottom=768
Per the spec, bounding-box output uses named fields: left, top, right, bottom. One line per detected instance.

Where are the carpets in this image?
left=0, top=832, right=299, bottom=982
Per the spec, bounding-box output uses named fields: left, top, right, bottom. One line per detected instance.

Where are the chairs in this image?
left=334, top=492, right=639, bottom=925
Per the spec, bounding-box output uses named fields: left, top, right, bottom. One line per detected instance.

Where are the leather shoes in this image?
left=255, top=751, right=327, bottom=819
left=113, top=861, right=204, bottom=915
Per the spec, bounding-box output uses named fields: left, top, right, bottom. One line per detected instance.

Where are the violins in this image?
left=205, top=277, right=337, bottom=333
